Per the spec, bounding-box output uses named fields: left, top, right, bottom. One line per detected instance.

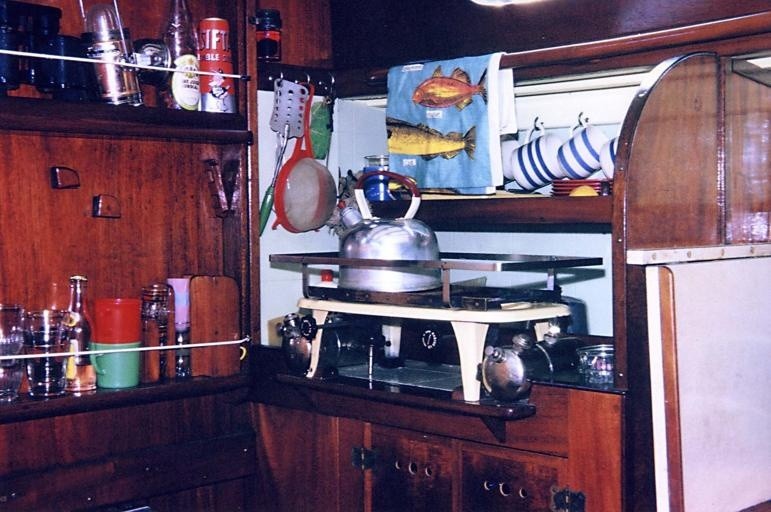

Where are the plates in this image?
left=549, top=177, right=614, bottom=197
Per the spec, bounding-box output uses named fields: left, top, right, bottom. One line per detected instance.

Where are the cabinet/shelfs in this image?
left=0, top=1, right=261, bottom=512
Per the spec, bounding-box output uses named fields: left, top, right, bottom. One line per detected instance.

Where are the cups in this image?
left=0, top=304, right=66, bottom=402
left=0, top=1, right=77, bottom=94
left=83, top=296, right=141, bottom=390
left=576, top=344, right=614, bottom=383
left=508, top=119, right=619, bottom=190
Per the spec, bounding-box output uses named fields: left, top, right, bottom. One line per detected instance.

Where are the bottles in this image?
left=164, top=1, right=200, bottom=112
left=317, top=268, right=337, bottom=290
left=141, top=282, right=174, bottom=383
left=83, top=5, right=144, bottom=111
left=60, top=273, right=96, bottom=393
left=255, top=9, right=283, bottom=62
left=196, top=16, right=237, bottom=112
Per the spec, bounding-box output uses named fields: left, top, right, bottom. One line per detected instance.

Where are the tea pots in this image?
left=337, top=171, right=441, bottom=294
